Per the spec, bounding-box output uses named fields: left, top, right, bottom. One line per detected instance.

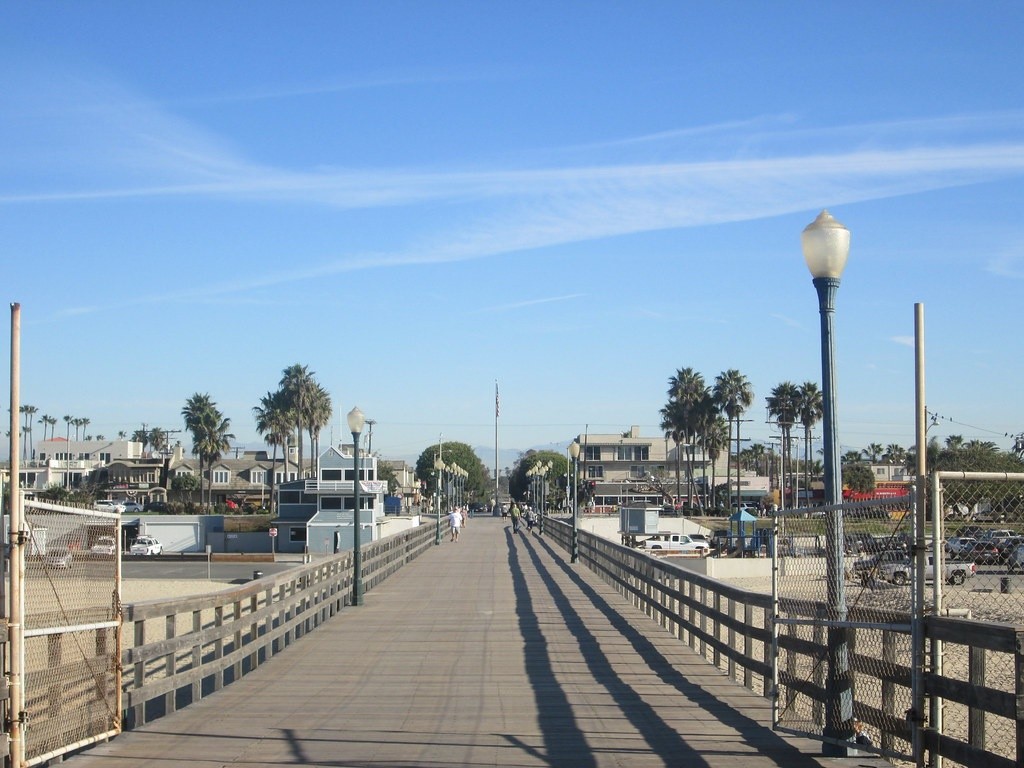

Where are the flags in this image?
left=496, top=385, right=499, bottom=417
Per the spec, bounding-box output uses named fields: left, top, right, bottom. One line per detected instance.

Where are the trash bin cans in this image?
left=254, top=570, right=264, bottom=579
left=1001, top=577, right=1011, bottom=593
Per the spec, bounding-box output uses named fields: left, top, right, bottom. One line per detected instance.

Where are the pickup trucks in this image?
left=640, top=534, right=709, bottom=554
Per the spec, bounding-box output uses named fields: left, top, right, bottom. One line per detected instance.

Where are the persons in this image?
left=501, top=503, right=509, bottom=523
left=757, top=498, right=766, bottom=518
left=510, top=503, right=521, bottom=534
left=448, top=506, right=463, bottom=543
left=460, top=508, right=467, bottom=528
left=524, top=506, right=535, bottom=529
left=671, top=504, right=682, bottom=517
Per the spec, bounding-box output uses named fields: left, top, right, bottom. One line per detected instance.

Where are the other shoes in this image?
left=450, top=538, right=458, bottom=542
left=513, top=529, right=518, bottom=534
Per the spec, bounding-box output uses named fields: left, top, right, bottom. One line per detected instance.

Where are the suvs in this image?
left=93, top=499, right=128, bottom=514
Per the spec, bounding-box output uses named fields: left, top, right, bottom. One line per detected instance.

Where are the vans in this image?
left=731, top=500, right=766, bottom=517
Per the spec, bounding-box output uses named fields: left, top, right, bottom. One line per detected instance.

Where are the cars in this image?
left=122, top=501, right=143, bottom=513
left=843, top=505, right=1024, bottom=587
left=91, top=536, right=117, bottom=555
left=143, top=501, right=168, bottom=514
left=709, top=529, right=734, bottom=549
left=130, top=537, right=163, bottom=556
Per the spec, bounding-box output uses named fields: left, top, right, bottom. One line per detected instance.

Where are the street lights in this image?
left=568, top=440, right=580, bottom=564
left=346, top=406, right=364, bottom=607
left=433, top=458, right=443, bottom=544
left=525, top=460, right=552, bottom=536
left=444, top=463, right=468, bottom=516
left=800, top=207, right=859, bottom=761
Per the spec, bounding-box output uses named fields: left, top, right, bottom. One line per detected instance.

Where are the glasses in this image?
left=454, top=509, right=457, bottom=510
